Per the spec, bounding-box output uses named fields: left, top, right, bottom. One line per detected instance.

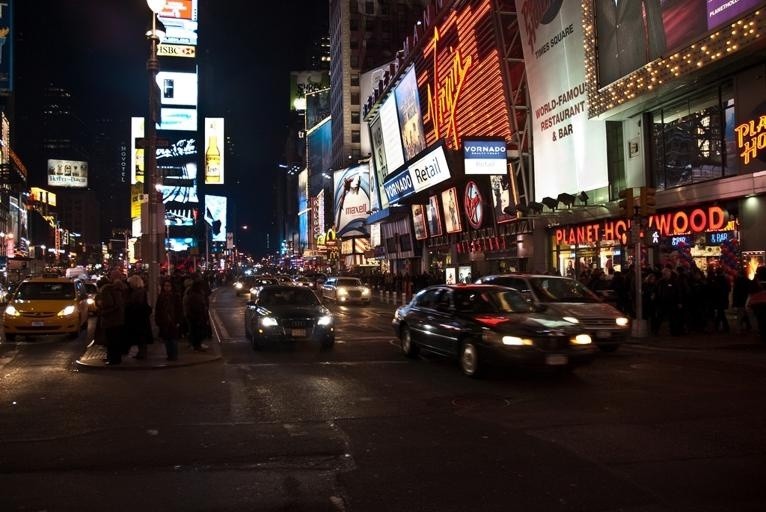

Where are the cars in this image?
left=3, top=273, right=91, bottom=342
left=233, top=275, right=317, bottom=300
left=475, top=274, right=631, bottom=354
left=0, top=284, right=8, bottom=304
left=321, top=277, right=373, bottom=306
left=392, top=283, right=599, bottom=378
left=83, top=283, right=101, bottom=313
left=245, top=284, right=336, bottom=354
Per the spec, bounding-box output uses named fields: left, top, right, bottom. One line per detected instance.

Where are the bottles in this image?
left=205, top=123, right=220, bottom=183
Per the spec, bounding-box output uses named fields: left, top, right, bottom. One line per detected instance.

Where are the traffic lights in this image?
left=642, top=189, right=658, bottom=217
left=618, top=187, right=635, bottom=220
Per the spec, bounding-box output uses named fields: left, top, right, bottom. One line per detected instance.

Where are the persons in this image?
left=289, top=250, right=766, bottom=338
left=0, top=262, right=238, bottom=370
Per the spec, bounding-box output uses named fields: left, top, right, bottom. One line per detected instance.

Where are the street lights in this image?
left=144, top=0, right=168, bottom=334
left=0, top=232, right=13, bottom=256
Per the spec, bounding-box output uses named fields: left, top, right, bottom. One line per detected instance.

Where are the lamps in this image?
left=503, top=190, right=591, bottom=218
